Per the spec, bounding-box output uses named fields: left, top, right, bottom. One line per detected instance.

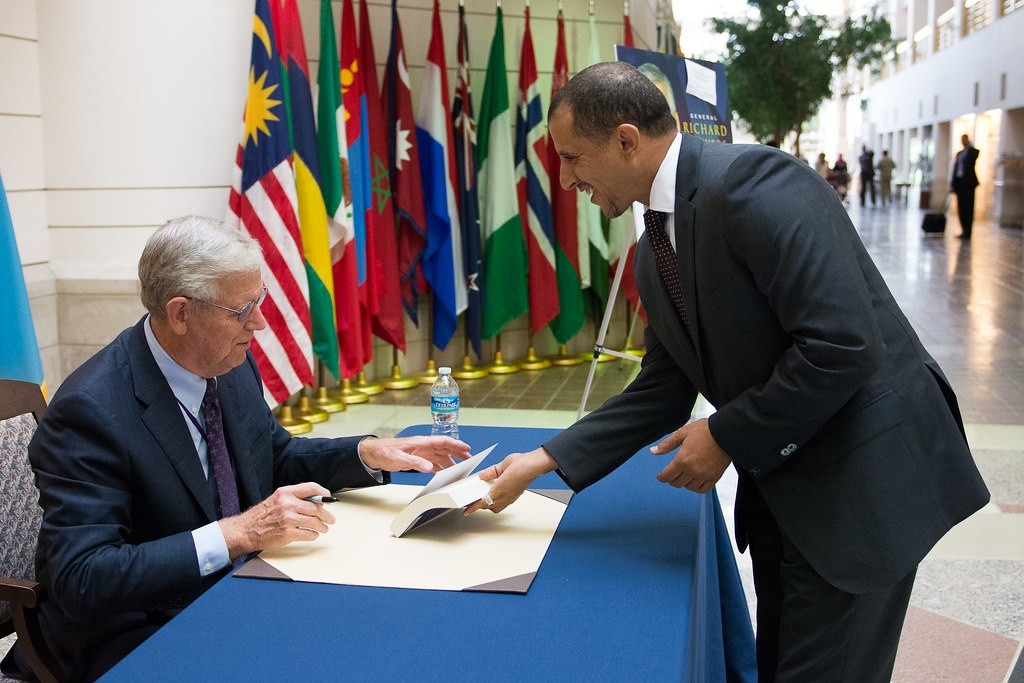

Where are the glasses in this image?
left=178, top=285, right=268, bottom=322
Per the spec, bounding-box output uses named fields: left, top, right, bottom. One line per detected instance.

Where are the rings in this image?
left=483, top=496, right=494, bottom=507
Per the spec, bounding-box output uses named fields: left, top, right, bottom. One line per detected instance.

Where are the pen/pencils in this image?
left=305, top=496, right=341, bottom=503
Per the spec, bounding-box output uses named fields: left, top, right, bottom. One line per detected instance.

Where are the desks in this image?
left=95, top=424, right=757, bottom=682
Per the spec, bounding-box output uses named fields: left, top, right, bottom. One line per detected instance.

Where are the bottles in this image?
left=430, top=367, right=459, bottom=441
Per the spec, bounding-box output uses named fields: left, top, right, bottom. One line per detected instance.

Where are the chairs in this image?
left=0, top=376, right=62, bottom=683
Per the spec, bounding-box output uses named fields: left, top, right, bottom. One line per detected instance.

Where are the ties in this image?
left=203, top=376, right=239, bottom=521
left=644, top=207, right=691, bottom=340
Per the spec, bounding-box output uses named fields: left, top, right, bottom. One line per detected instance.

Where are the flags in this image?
left=224, top=0, right=686, bottom=412
left=0, top=180, right=48, bottom=405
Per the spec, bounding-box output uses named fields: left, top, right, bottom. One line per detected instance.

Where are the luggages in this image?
left=922, top=191, right=952, bottom=232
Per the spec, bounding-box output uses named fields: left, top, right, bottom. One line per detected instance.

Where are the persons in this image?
left=877, top=151, right=896, bottom=207
left=464, top=61, right=991, bottom=683
left=858, top=145, right=876, bottom=207
left=815, top=152, right=848, bottom=201
left=950, top=134, right=980, bottom=240
left=26, top=213, right=472, bottom=683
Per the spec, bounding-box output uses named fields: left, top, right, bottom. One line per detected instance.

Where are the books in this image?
left=389, top=444, right=496, bottom=538
